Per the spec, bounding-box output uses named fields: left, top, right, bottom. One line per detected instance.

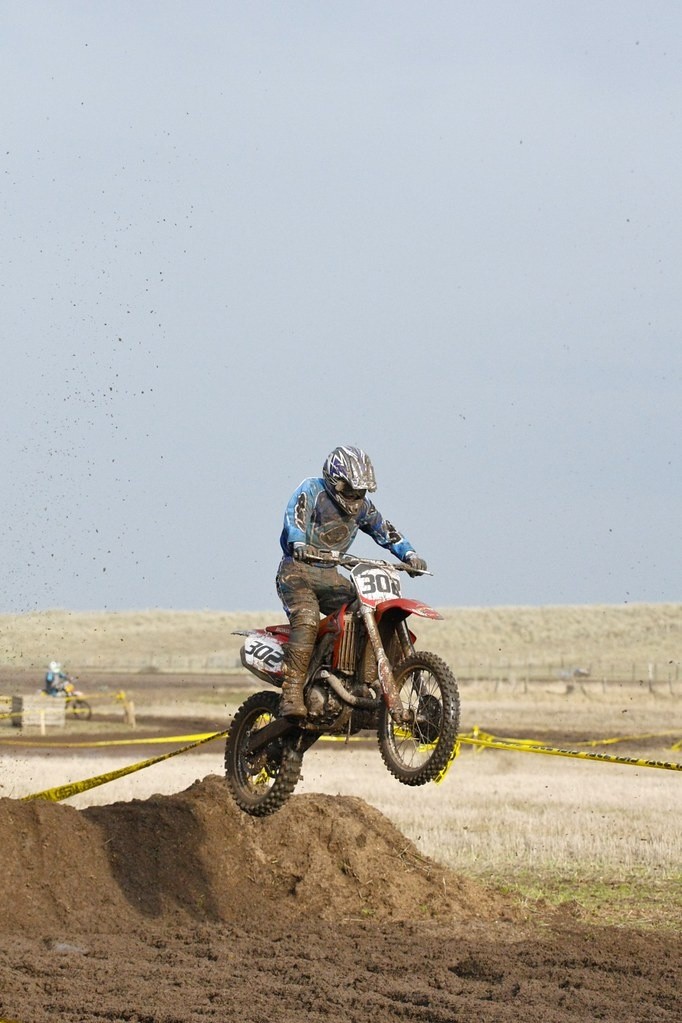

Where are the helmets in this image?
left=321, top=444, right=379, bottom=516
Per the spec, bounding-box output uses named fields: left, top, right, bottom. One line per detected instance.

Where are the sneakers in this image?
left=277, top=675, right=307, bottom=720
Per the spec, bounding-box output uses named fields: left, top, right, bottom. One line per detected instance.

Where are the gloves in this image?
left=404, top=557, right=427, bottom=579
left=294, top=544, right=318, bottom=565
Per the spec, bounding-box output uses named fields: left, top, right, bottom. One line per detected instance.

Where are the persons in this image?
left=275, top=445, right=428, bottom=720
left=46, top=660, right=73, bottom=698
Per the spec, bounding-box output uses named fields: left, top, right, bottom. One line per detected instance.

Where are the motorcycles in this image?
left=38, top=675, right=92, bottom=720
left=224, top=547, right=461, bottom=817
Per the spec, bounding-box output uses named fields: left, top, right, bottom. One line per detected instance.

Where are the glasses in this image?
left=339, top=486, right=367, bottom=500
